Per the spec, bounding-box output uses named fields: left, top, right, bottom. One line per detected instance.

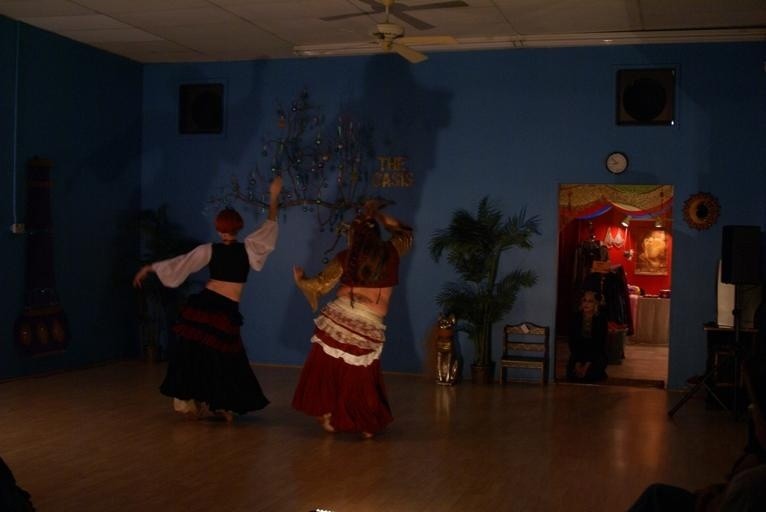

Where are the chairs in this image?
left=501, top=322, right=552, bottom=384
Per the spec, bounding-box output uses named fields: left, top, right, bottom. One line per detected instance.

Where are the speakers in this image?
left=721, top=224, right=764, bottom=288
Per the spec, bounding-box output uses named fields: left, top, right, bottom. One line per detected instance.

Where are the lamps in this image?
left=621, top=215, right=664, bottom=230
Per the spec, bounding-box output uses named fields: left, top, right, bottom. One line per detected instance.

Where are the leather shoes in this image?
left=315, top=411, right=376, bottom=439
left=197, top=399, right=236, bottom=427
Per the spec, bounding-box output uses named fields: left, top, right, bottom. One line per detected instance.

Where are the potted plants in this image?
left=425, top=193, right=539, bottom=383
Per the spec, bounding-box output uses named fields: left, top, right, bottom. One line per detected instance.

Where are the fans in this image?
left=294, top=3, right=458, bottom=66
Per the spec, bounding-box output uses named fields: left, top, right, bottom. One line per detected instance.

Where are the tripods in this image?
left=666, top=286, right=756, bottom=420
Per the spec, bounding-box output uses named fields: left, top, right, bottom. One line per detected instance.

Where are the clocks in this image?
left=606, top=151, right=631, bottom=175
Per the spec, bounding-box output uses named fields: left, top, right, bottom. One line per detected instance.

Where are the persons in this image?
left=624, top=335, right=766, bottom=511
left=130, top=177, right=285, bottom=425
left=289, top=198, right=415, bottom=443
left=564, top=291, right=612, bottom=383
